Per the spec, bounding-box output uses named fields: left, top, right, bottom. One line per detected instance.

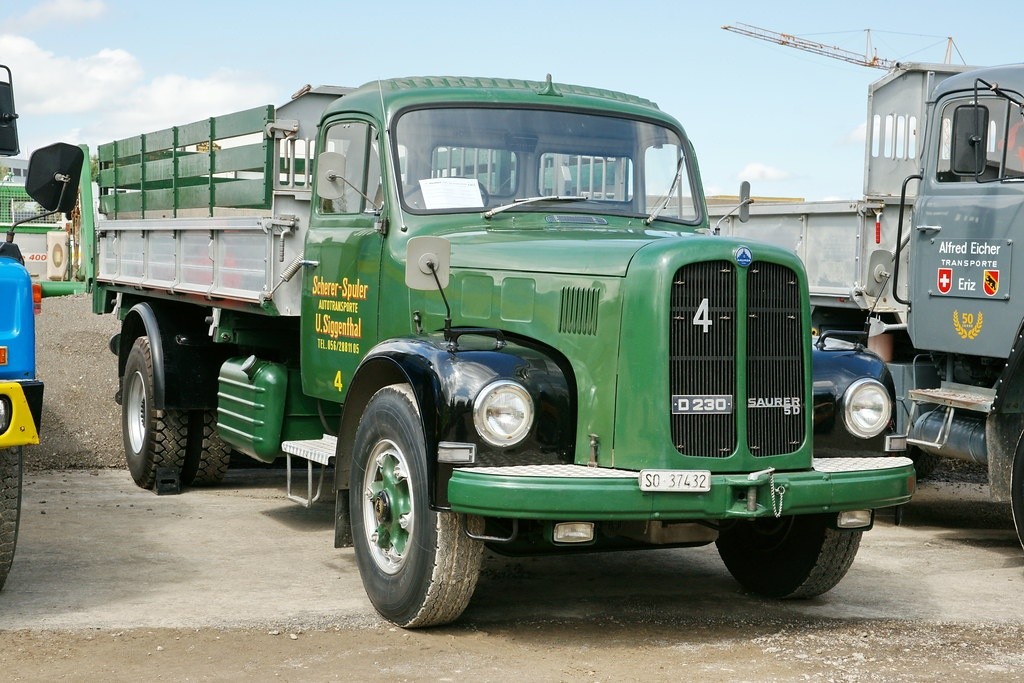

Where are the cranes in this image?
left=722, top=21, right=970, bottom=72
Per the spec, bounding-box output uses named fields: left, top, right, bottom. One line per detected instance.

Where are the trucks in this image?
left=75, top=76, right=917, bottom=631
left=642, top=63, right=1023, bottom=553
left=0, top=64, right=83, bottom=593
